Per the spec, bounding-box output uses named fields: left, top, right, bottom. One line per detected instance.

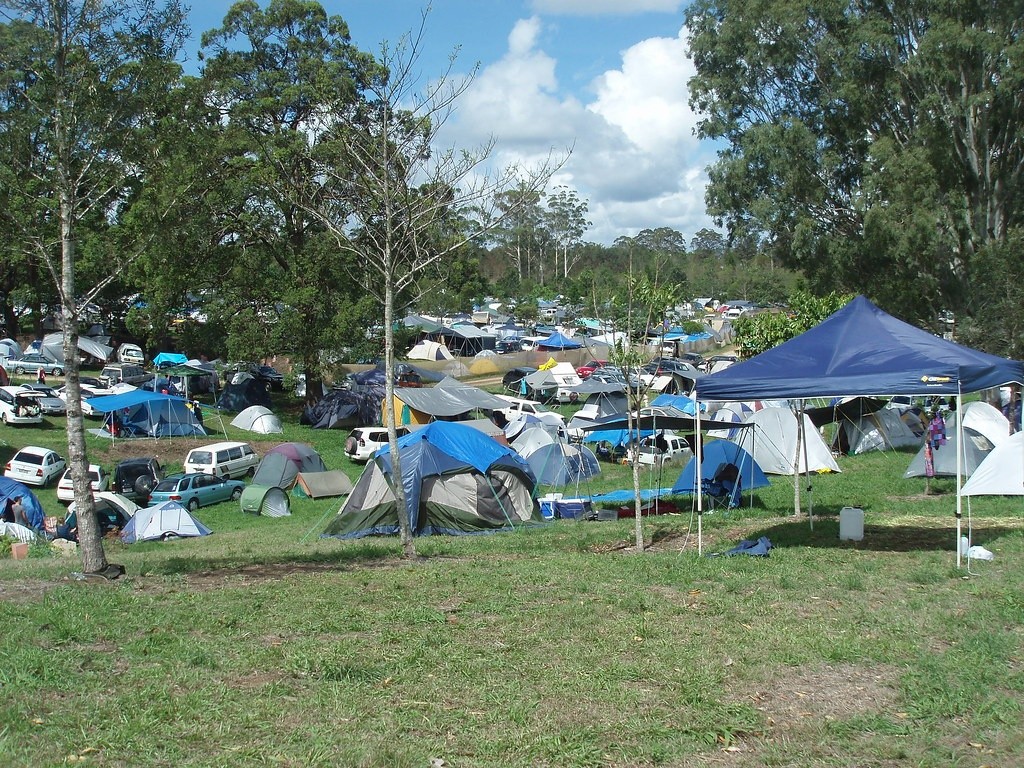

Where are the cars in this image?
left=399, top=372, right=423, bottom=388
left=223, top=363, right=284, bottom=393
left=20, top=382, right=67, bottom=416
left=2, top=446, right=67, bottom=489
left=6, top=353, right=64, bottom=376
left=51, top=383, right=114, bottom=417
left=79, top=377, right=105, bottom=390
left=57, top=464, right=112, bottom=505
left=493, top=332, right=737, bottom=417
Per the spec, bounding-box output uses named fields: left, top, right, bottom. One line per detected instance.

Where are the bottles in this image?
left=839, top=505, right=863, bottom=541
left=961, top=534, right=968, bottom=555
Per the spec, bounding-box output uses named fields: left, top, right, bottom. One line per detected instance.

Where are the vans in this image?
left=115, top=342, right=146, bottom=368
left=0, top=385, right=48, bottom=426
left=185, top=441, right=259, bottom=480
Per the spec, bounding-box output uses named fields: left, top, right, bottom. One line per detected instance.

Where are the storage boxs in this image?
left=538, top=494, right=592, bottom=521
left=598, top=509, right=618, bottom=521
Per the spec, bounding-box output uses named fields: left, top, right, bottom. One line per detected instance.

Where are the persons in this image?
left=37, top=366, right=46, bottom=384
left=193, top=404, right=203, bottom=429
left=122, top=408, right=136, bottom=437
left=11, top=496, right=30, bottom=529
left=597, top=440, right=612, bottom=460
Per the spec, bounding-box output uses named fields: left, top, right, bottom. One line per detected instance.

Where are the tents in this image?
left=0, top=294, right=1024, bottom=570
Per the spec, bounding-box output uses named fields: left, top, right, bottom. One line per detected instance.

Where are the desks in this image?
left=122, top=426, right=139, bottom=439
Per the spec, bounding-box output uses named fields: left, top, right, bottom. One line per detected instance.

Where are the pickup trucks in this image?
left=493, top=398, right=567, bottom=429
left=627, top=434, right=693, bottom=468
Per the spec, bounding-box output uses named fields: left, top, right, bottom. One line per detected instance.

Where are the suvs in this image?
left=111, top=458, right=166, bottom=507
left=99, top=364, right=154, bottom=388
left=344, top=427, right=390, bottom=461
left=147, top=472, right=246, bottom=513
